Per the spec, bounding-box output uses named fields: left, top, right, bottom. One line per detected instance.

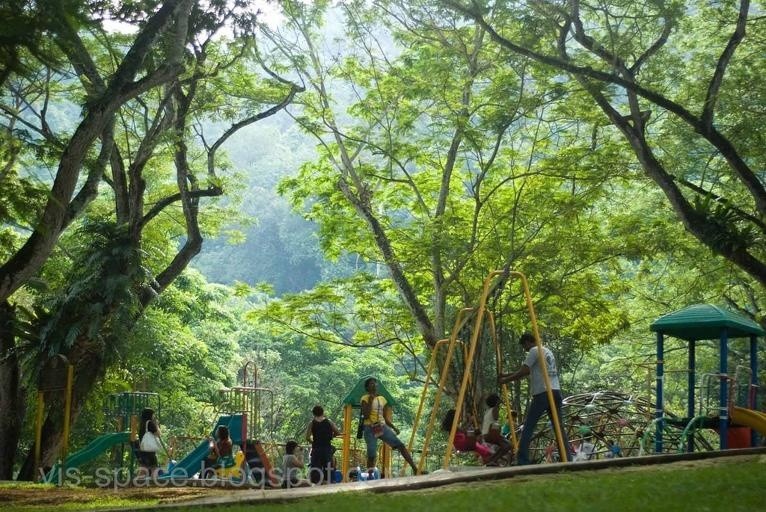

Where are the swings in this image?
left=449, top=290, right=504, bottom=462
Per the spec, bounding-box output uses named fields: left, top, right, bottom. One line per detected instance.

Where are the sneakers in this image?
left=415, top=471, right=428, bottom=475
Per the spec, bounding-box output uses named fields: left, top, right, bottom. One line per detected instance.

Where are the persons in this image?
left=640, top=303, right=766, bottom=458
left=304, top=406, right=340, bottom=482
left=604, top=440, right=624, bottom=457
left=480, top=394, right=513, bottom=467
left=570, top=434, right=586, bottom=462
left=348, top=468, right=361, bottom=481
left=502, top=409, right=518, bottom=438
left=441, top=408, right=498, bottom=466
left=580, top=431, right=597, bottom=460
left=359, top=377, right=429, bottom=476
left=197, top=424, right=233, bottom=479
left=496, top=333, right=574, bottom=465
left=280, top=440, right=309, bottom=487
left=35, top=367, right=164, bottom=486
left=133, top=408, right=161, bottom=478
left=153, top=361, right=283, bottom=488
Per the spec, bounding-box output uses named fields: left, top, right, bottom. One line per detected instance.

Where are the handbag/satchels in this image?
left=140, top=432, right=160, bottom=452
left=371, top=422, right=383, bottom=438
left=357, top=407, right=363, bottom=438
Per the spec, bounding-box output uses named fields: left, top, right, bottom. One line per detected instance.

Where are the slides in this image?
left=36, top=431, right=130, bottom=485
left=145, top=415, right=278, bottom=489
left=730, top=405, right=766, bottom=438
